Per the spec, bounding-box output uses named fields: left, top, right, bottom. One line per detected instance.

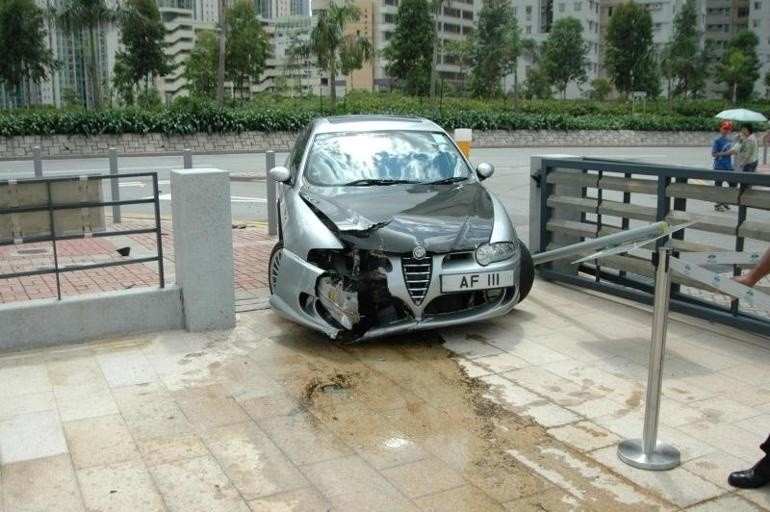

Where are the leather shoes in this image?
left=728, top=465, right=769, bottom=488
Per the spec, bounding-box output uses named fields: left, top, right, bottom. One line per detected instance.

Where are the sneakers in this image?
left=714, top=202, right=731, bottom=212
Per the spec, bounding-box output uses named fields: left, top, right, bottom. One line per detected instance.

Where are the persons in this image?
left=710, top=121, right=740, bottom=213
left=727, top=433, right=770, bottom=488
left=715, top=244, right=770, bottom=301
left=737, top=122, right=759, bottom=191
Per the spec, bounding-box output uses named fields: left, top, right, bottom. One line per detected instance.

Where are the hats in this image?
left=719, top=121, right=733, bottom=130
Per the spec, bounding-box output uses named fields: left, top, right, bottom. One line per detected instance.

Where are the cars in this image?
left=268, top=114, right=534, bottom=345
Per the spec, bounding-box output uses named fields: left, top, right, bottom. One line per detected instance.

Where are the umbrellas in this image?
left=713, top=108, right=769, bottom=122
